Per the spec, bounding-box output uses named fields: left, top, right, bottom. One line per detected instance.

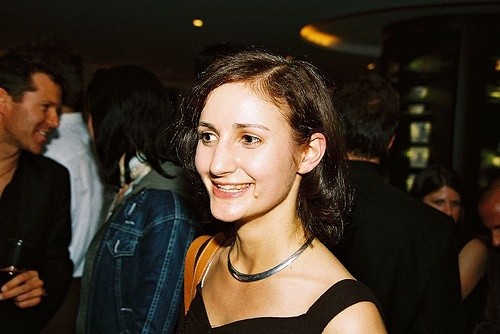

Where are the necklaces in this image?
left=227, top=230, right=316, bottom=283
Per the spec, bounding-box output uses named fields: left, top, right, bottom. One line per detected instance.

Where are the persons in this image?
left=0, top=42, right=500, bottom=334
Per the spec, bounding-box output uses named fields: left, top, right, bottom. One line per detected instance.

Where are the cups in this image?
left=0, top=239, right=27, bottom=293
